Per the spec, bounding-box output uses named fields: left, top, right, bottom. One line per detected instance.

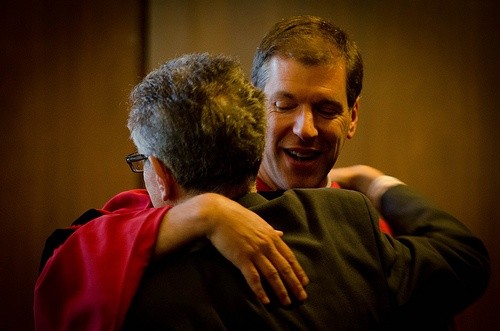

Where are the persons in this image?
left=93, top=51, right=490, bottom=331
left=33, top=16, right=395, bottom=329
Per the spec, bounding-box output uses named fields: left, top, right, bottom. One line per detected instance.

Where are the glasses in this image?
left=125, top=151, right=148, bottom=173
